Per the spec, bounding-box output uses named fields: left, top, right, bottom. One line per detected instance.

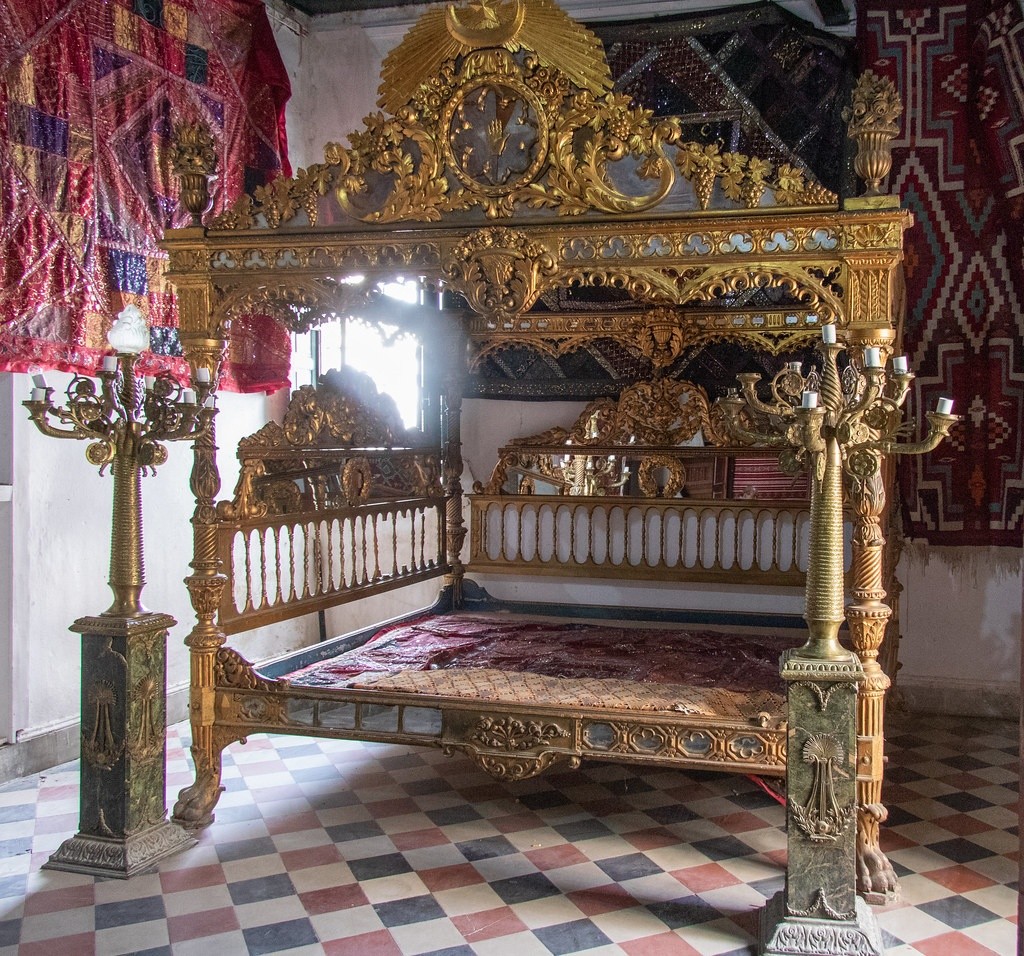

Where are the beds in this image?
left=156, top=0, right=917, bottom=890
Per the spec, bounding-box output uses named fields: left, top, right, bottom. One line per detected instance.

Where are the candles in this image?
left=144, top=375, right=157, bottom=389
left=183, top=391, right=197, bottom=403
left=32, top=374, right=47, bottom=387
left=865, top=347, right=881, bottom=366
left=104, top=356, right=117, bottom=372
left=822, top=325, right=838, bottom=344
left=936, top=397, right=954, bottom=415
left=31, top=389, right=46, bottom=401
left=893, top=355, right=906, bottom=373
left=195, top=367, right=211, bottom=382
left=801, top=391, right=818, bottom=407
